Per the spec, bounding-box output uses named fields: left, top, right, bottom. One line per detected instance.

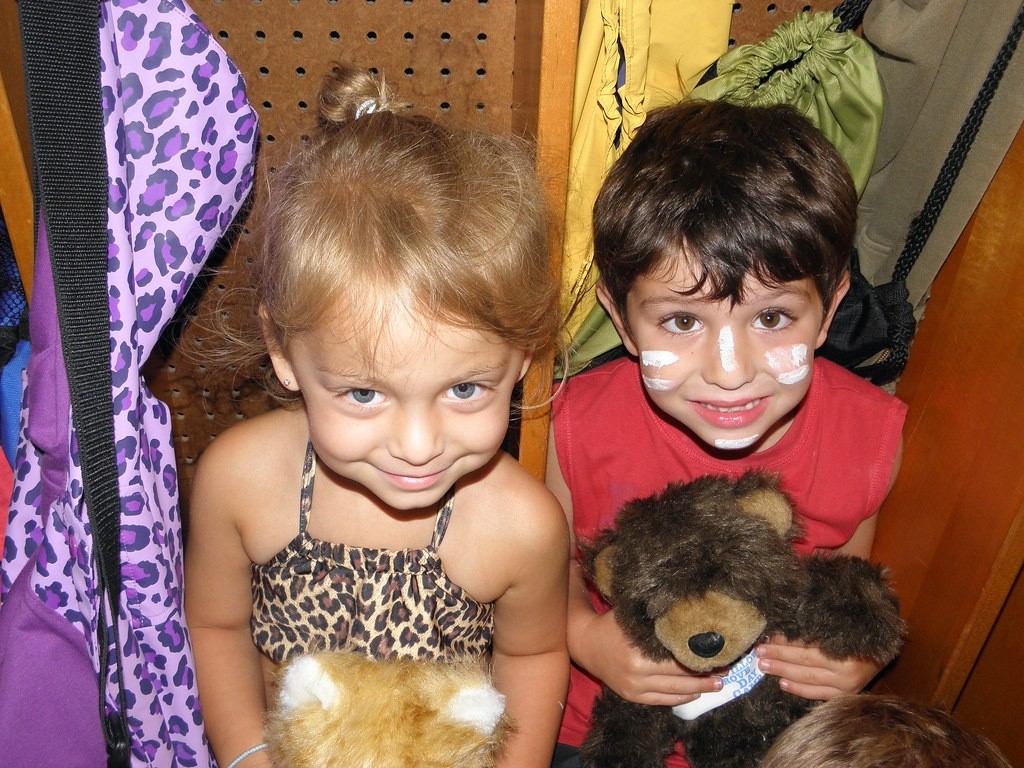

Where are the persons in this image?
left=758, top=695, right=1012, bottom=768
left=183, top=58, right=572, bottom=768
left=544, top=97, right=908, bottom=768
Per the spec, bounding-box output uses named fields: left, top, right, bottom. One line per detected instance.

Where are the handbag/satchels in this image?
left=813, top=246, right=918, bottom=384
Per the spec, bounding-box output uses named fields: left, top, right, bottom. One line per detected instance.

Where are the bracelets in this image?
left=227, top=743, right=271, bottom=768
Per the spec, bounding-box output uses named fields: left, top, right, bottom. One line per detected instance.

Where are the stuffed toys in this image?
left=572, top=465, right=906, bottom=768
